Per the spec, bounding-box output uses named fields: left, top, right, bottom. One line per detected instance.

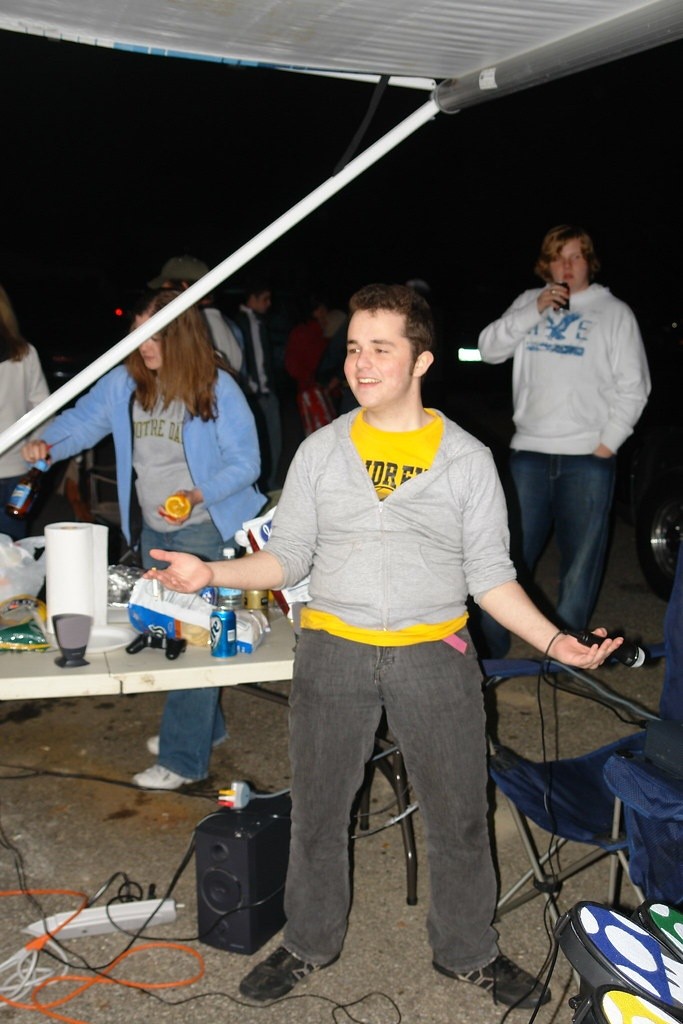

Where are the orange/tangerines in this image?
left=165, top=495, right=191, bottom=518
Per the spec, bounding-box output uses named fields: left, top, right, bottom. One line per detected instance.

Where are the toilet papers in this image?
left=43, top=521, right=110, bottom=637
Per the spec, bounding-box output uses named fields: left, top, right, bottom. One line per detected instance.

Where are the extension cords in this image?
left=22, top=899, right=177, bottom=943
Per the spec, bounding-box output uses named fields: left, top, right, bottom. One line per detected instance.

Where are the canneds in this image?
left=552, top=281, right=570, bottom=313
left=199, top=585, right=220, bottom=606
left=243, top=589, right=270, bottom=618
left=210, top=607, right=237, bottom=657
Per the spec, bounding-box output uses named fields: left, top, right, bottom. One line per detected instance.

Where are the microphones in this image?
left=567, top=629, right=645, bottom=669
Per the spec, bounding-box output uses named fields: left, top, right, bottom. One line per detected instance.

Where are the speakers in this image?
left=192, top=790, right=301, bottom=957
left=51, top=613, right=93, bottom=667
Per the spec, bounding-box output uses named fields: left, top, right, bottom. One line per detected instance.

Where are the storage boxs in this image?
left=247, top=519, right=314, bottom=618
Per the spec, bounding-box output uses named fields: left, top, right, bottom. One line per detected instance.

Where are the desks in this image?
left=0, top=601, right=417, bottom=905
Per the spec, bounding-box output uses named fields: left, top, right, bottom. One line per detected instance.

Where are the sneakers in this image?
left=432, top=951, right=551, bottom=1009
left=238, top=946, right=340, bottom=1000
left=133, top=765, right=192, bottom=790
left=147, top=735, right=161, bottom=756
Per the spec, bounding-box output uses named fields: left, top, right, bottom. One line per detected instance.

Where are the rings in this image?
left=549, top=288, right=556, bottom=295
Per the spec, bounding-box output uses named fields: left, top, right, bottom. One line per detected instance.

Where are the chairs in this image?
left=481, top=548, right=683, bottom=988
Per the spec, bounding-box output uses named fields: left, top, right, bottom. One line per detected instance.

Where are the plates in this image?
left=85, top=626, right=137, bottom=652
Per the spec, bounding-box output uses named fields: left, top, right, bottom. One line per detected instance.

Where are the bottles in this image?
left=8, top=454, right=51, bottom=520
left=217, top=546, right=242, bottom=610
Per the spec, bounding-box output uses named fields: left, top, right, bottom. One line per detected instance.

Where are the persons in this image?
left=58, top=257, right=358, bottom=571
left=149, top=283, right=624, bottom=1009
left=21, top=287, right=267, bottom=789
left=0, top=289, right=57, bottom=540
left=478, top=227, right=652, bottom=659
